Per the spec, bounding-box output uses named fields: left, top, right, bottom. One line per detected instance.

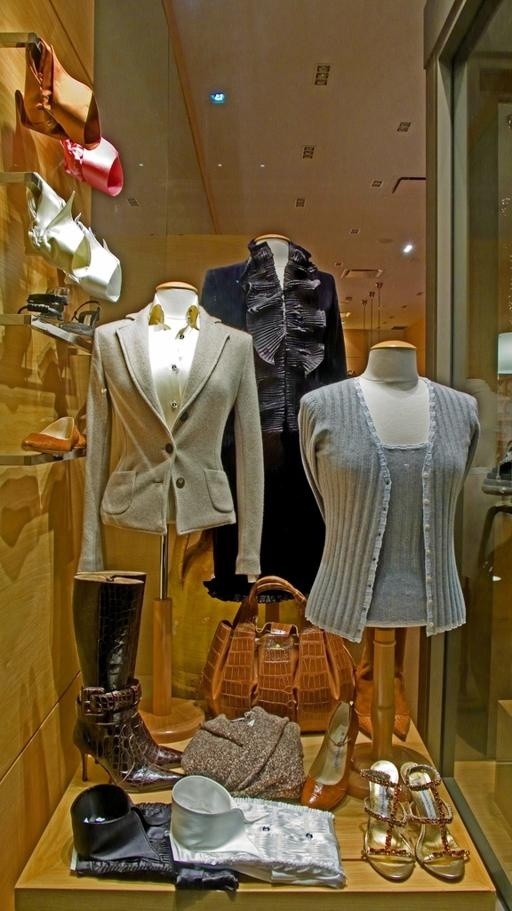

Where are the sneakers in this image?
left=481, top=447, right=512, bottom=496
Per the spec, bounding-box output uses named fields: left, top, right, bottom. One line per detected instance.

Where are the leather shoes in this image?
left=353, top=704, right=411, bottom=741
left=21, top=405, right=87, bottom=456
left=300, top=700, right=359, bottom=811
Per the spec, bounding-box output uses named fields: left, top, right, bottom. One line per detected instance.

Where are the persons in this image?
left=78, top=273, right=267, bottom=714
left=198, top=232, right=354, bottom=623
left=295, top=332, right=482, bottom=766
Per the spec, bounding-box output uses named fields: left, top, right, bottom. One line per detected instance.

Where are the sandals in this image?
left=26, top=286, right=71, bottom=321
left=58, top=299, right=103, bottom=337
left=359, top=759, right=471, bottom=882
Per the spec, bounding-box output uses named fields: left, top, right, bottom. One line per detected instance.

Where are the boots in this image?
left=72, top=570, right=186, bottom=795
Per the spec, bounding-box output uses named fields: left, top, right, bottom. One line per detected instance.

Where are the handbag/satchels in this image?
left=197, top=619, right=357, bottom=733
